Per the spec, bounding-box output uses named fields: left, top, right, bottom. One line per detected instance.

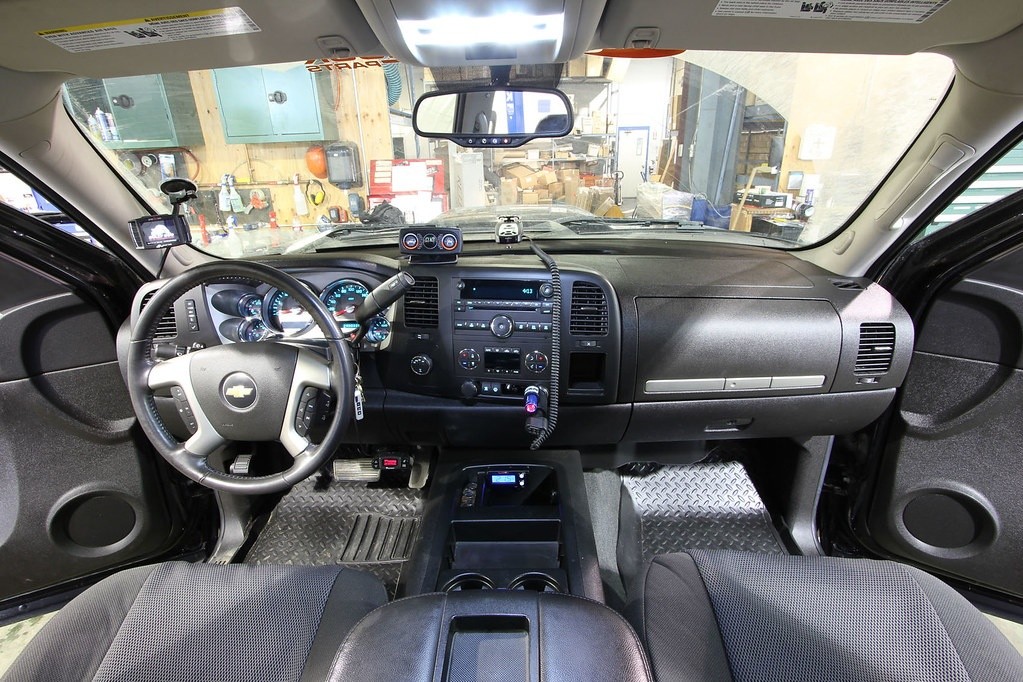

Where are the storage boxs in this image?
left=421, top=54, right=624, bottom=220
left=654, top=58, right=813, bottom=243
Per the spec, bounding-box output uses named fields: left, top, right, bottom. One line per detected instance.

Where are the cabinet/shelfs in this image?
left=60, top=71, right=206, bottom=151
left=209, top=58, right=340, bottom=145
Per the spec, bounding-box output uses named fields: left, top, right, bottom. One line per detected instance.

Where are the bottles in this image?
left=293, top=173, right=308, bottom=215
left=219, top=173, right=243, bottom=213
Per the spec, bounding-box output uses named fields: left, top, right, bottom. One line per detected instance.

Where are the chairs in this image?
left=634, top=549, right=1023, bottom=682
left=0, top=558, right=388, bottom=680
